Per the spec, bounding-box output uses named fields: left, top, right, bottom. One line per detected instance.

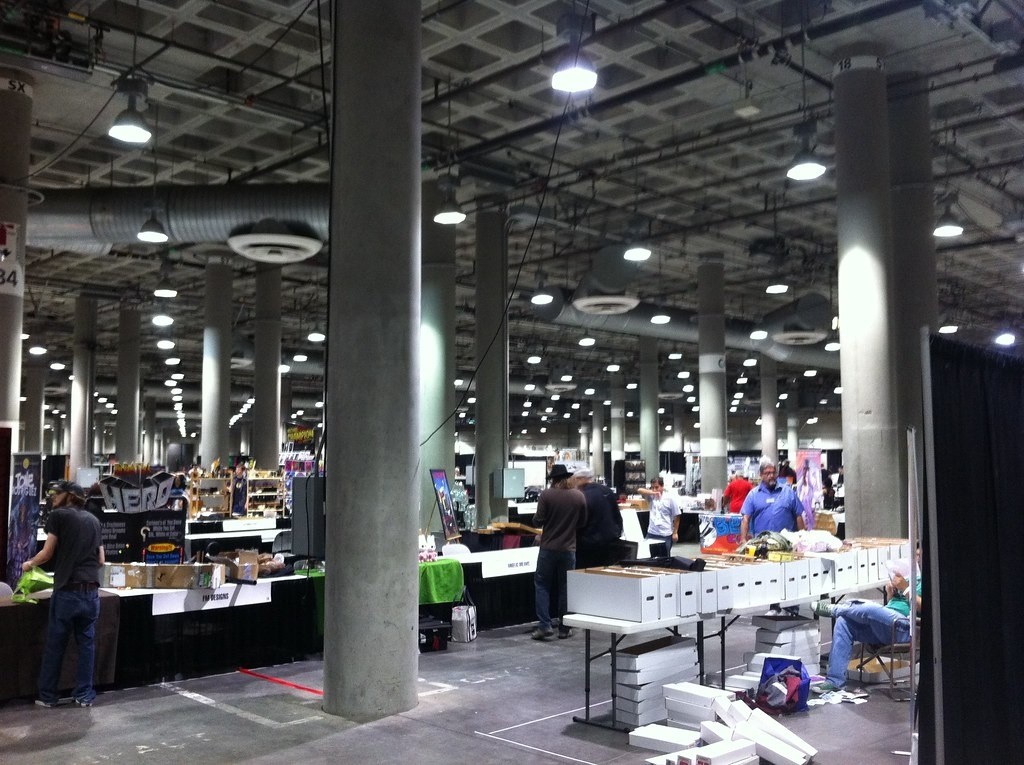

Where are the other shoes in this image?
left=765, top=610, right=779, bottom=615
left=557, top=629, right=573, bottom=640
left=532, top=624, right=554, bottom=638
left=779, top=608, right=790, bottom=616
left=33, top=699, right=56, bottom=708
left=74, top=698, right=93, bottom=708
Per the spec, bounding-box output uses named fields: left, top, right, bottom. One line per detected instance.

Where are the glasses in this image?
left=762, top=470, right=778, bottom=476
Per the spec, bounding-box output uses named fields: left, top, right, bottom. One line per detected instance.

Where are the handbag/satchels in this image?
left=451, top=585, right=478, bottom=643
left=756, top=656, right=809, bottom=716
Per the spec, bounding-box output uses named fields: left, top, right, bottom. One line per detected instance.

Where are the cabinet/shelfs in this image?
left=248, top=477, right=286, bottom=517
left=190, top=478, right=233, bottom=518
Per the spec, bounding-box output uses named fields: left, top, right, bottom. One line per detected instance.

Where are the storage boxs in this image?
left=567, top=536, right=910, bottom=622
left=613, top=613, right=919, bottom=765
left=99, top=547, right=260, bottom=589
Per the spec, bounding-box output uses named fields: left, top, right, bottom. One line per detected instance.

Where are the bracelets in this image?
left=903, top=587, right=909, bottom=596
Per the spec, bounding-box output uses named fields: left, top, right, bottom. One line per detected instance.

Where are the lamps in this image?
left=623, top=143, right=651, bottom=262
left=530, top=222, right=554, bottom=305
left=551, top=0, right=597, bottom=94
left=932, top=113, right=963, bottom=237
left=650, top=240, right=671, bottom=325
left=432, top=72, right=467, bottom=225
left=764, top=192, right=789, bottom=294
left=106, top=0, right=185, bottom=387
left=786, top=0, right=826, bottom=180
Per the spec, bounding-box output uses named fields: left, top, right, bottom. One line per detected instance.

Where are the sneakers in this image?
left=812, top=679, right=839, bottom=695
left=810, top=602, right=836, bottom=618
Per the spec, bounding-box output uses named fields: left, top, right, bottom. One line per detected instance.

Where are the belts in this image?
left=60, top=583, right=98, bottom=592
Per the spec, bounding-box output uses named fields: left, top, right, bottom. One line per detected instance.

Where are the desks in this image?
left=562, top=577, right=906, bottom=733
left=440, top=536, right=666, bottom=633
left=0, top=572, right=318, bottom=701
left=293, top=560, right=464, bottom=637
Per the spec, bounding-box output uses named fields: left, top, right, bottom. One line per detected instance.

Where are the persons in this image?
left=722, top=471, right=754, bottom=514
left=532, top=465, right=587, bottom=639
left=811, top=541, right=922, bottom=694
left=575, top=469, right=623, bottom=568
left=740, top=462, right=806, bottom=545
left=779, top=459, right=844, bottom=510
left=22, top=482, right=104, bottom=707
left=638, top=477, right=681, bottom=557
left=226, top=464, right=247, bottom=516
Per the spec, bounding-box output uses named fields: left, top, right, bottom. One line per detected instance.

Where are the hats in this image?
left=572, top=468, right=593, bottom=480
left=547, top=464, right=574, bottom=481
left=50, top=481, right=86, bottom=502
